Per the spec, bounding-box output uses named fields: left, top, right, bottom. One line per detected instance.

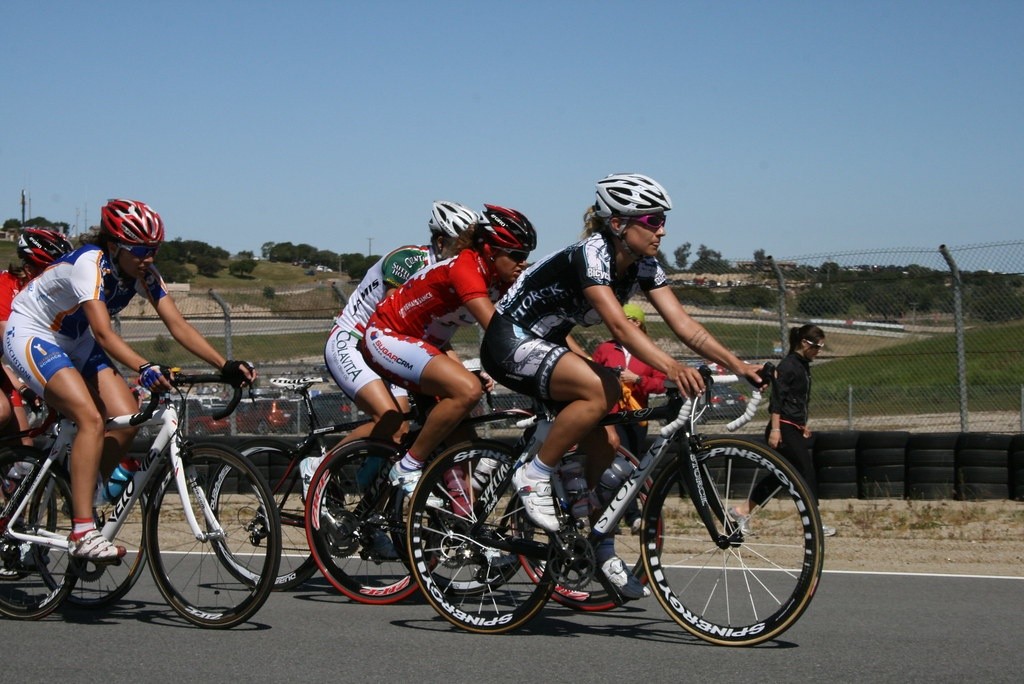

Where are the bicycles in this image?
left=206, top=366, right=665, bottom=611
left=406, top=362, right=825, bottom=647
left=0, top=367, right=282, bottom=629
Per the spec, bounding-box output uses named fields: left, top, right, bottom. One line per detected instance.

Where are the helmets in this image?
left=594, top=173, right=672, bottom=220
left=476, top=203, right=537, bottom=251
left=15, top=227, right=73, bottom=268
left=101, top=199, right=164, bottom=247
left=621, top=304, right=645, bottom=324
left=428, top=200, right=480, bottom=240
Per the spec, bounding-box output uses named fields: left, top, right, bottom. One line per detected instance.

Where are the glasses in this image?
left=110, top=239, right=158, bottom=257
left=613, top=213, right=667, bottom=228
left=489, top=243, right=529, bottom=261
left=805, top=339, right=826, bottom=351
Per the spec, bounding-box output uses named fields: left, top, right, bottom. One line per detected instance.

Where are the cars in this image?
left=317, top=266, right=333, bottom=272
left=696, top=385, right=747, bottom=426
left=665, top=278, right=745, bottom=287
left=140, top=386, right=534, bottom=436
left=695, top=359, right=746, bottom=383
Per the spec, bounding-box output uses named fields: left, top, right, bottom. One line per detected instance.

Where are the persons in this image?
left=726, top=325, right=836, bottom=538
left=0, top=229, right=140, bottom=528
left=3, top=200, right=257, bottom=560
left=323, top=173, right=778, bottom=598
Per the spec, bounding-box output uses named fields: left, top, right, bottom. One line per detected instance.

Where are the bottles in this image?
left=560, top=458, right=590, bottom=518
left=590, top=456, right=633, bottom=508
left=0, top=457, right=34, bottom=504
left=443, top=462, right=471, bottom=517
left=102, top=457, right=139, bottom=502
left=471, top=458, right=498, bottom=490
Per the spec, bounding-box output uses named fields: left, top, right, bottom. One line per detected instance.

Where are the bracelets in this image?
left=636, top=378, right=640, bottom=383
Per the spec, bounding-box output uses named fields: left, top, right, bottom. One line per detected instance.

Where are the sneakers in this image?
left=388, top=462, right=444, bottom=508
left=358, top=532, right=400, bottom=560
left=299, top=456, right=325, bottom=501
left=67, top=532, right=126, bottom=558
left=510, top=461, right=560, bottom=532
left=729, top=507, right=751, bottom=536
left=823, top=524, right=837, bottom=537
left=589, top=555, right=651, bottom=599
